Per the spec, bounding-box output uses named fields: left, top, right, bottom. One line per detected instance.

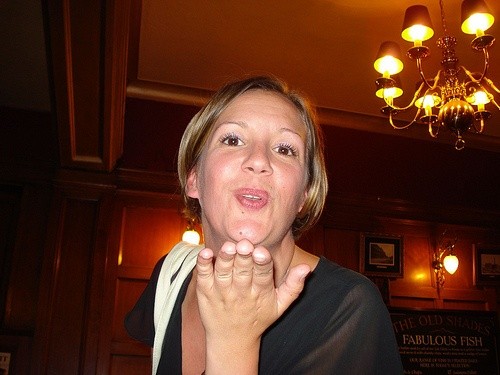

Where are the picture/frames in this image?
left=472, top=244, right=500, bottom=286
left=359, top=232, right=404, bottom=278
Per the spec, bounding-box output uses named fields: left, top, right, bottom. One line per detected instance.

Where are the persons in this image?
left=125, top=74, right=403, bottom=375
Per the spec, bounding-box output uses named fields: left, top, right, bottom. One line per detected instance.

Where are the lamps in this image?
left=373, top=0, right=500, bottom=151
left=432, top=242, right=459, bottom=302
